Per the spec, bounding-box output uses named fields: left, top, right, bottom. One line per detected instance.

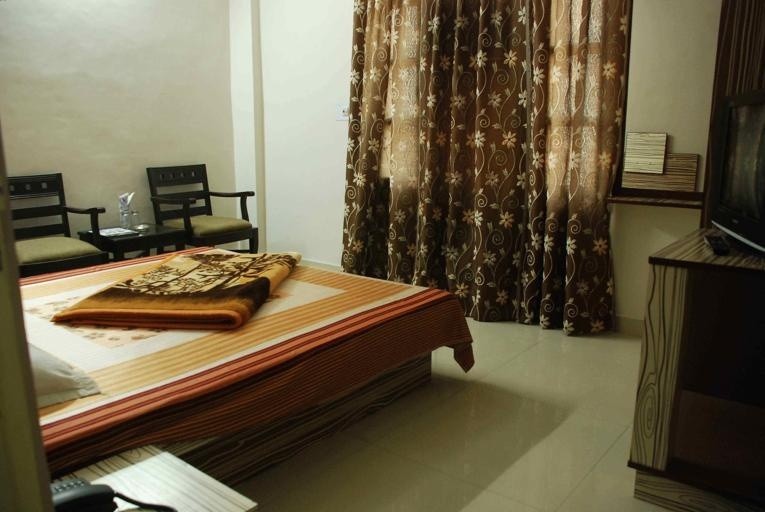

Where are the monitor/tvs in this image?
left=708, top=91, right=765, bottom=259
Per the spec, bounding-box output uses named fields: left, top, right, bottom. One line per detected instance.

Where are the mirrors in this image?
left=609, top=0, right=727, bottom=203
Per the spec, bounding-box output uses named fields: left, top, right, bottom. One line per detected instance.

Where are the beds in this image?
left=19, top=244, right=459, bottom=486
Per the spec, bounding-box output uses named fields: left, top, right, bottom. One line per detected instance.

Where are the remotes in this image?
left=704, top=235, right=730, bottom=256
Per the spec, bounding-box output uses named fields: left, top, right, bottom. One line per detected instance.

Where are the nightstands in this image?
left=46, top=442, right=260, bottom=512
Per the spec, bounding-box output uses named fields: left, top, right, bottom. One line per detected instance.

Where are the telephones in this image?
left=50, top=478, right=117, bottom=512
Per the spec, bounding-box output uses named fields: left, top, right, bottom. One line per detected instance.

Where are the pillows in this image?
left=24, top=338, right=101, bottom=411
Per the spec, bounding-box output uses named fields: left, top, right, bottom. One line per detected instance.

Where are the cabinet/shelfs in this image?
left=626, top=227, right=764, bottom=512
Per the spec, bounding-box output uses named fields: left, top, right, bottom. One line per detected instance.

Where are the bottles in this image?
left=120, top=210, right=141, bottom=229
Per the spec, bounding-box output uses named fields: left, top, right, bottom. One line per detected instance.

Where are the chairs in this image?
left=4, top=172, right=106, bottom=275
left=146, top=163, right=260, bottom=258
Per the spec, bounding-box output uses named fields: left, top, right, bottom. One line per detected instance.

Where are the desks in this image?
left=77, top=221, right=187, bottom=262
